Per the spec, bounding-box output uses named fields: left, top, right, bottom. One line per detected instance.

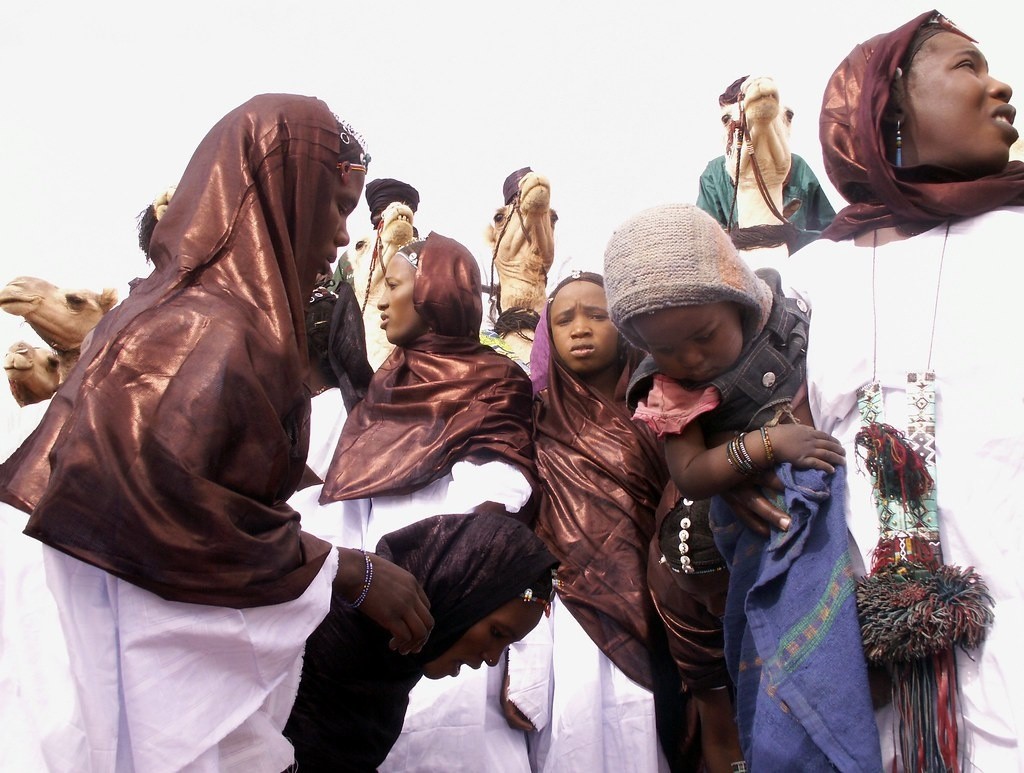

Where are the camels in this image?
left=357, top=200, right=418, bottom=362
left=718, top=72, right=794, bottom=271
left=0, top=275, right=119, bottom=408
left=487, top=173, right=559, bottom=367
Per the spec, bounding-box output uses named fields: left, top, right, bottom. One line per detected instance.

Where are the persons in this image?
left=720, top=9, right=1024, bottom=773
left=604, top=204, right=848, bottom=503
left=0, top=90, right=674, bottom=773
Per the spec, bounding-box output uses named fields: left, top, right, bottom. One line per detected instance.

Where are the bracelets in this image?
left=761, top=426, right=775, bottom=463
left=342, top=547, right=372, bottom=607
left=726, top=432, right=758, bottom=475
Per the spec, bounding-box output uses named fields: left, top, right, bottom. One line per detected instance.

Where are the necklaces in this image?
left=870, top=218, right=950, bottom=380
